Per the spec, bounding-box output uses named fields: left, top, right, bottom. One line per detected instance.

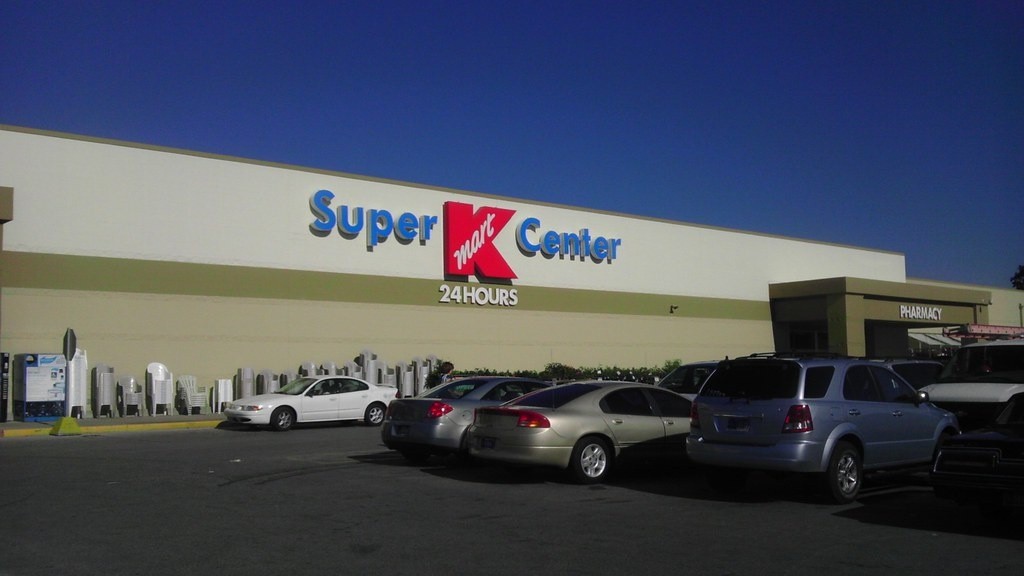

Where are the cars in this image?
left=916, top=339, right=1024, bottom=434
left=870, top=359, right=944, bottom=391
left=929, top=392, right=1024, bottom=520
left=467, top=380, right=693, bottom=484
left=655, top=363, right=718, bottom=401
left=224, top=375, right=401, bottom=432
left=380, top=376, right=554, bottom=462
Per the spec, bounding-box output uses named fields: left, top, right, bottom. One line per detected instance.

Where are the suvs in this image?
left=686, top=352, right=962, bottom=505
